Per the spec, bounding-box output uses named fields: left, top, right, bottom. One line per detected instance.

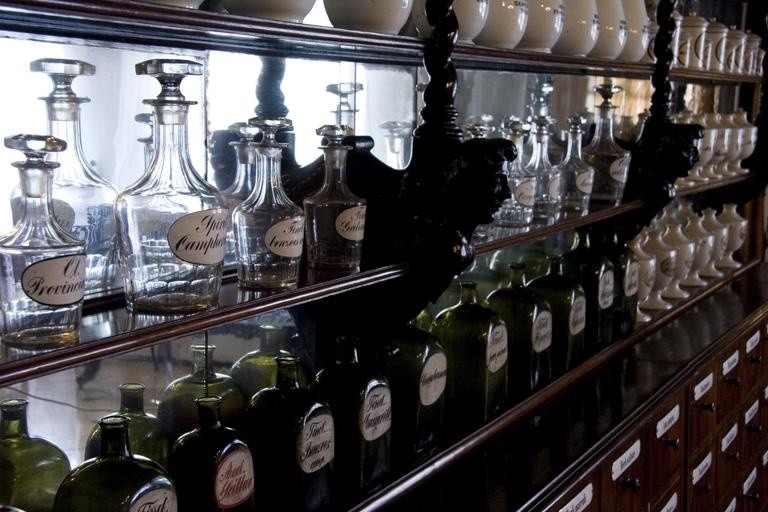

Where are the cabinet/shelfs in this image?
left=0, top=0, right=768, bottom=512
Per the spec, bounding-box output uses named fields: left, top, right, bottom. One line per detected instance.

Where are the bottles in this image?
left=2, top=0, right=764, bottom=511
